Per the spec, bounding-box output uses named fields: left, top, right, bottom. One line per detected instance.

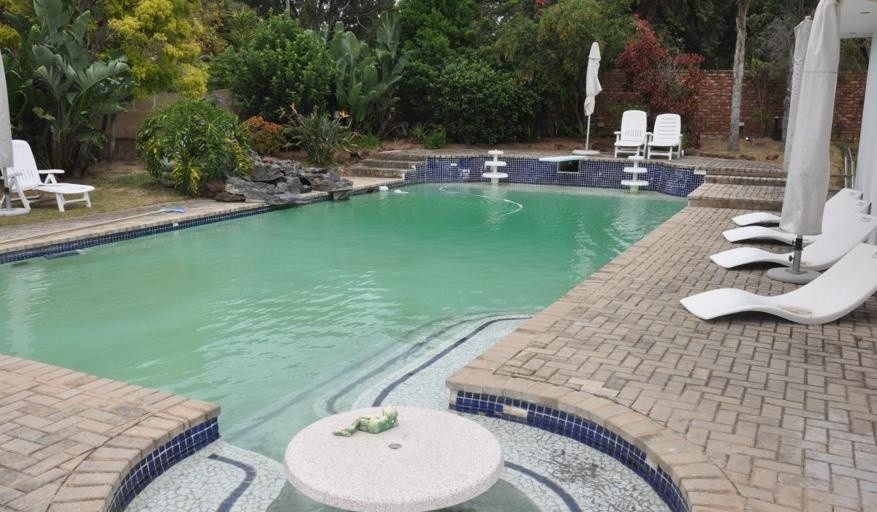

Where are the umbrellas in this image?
left=584, top=41, right=603, bottom=150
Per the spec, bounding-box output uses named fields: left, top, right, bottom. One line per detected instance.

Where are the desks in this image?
left=284, top=406, right=505, bottom=512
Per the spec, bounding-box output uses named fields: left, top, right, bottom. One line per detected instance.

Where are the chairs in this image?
left=1, top=139, right=96, bottom=213
left=731, top=188, right=863, bottom=227
left=678, top=242, right=877, bottom=325
left=613, top=109, right=647, bottom=159
left=646, top=113, right=683, bottom=161
left=721, top=200, right=871, bottom=247
left=709, top=213, right=877, bottom=269
